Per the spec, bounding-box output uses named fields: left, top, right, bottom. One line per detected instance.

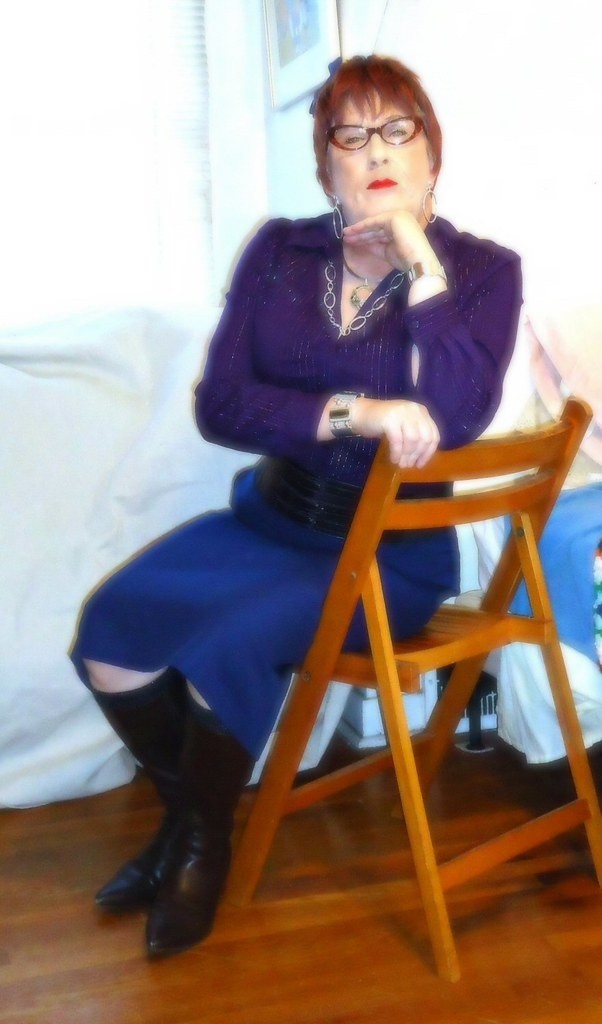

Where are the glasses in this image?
left=325, top=116, right=424, bottom=153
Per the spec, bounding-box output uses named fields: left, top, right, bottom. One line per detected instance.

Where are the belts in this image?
left=256, top=456, right=453, bottom=542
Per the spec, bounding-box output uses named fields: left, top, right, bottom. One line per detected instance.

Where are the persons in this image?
left=69, top=56, right=524, bottom=955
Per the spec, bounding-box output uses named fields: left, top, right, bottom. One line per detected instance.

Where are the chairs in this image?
left=217, top=395, right=602, bottom=981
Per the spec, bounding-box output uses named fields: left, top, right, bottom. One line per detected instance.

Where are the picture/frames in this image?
left=262, top=0, right=340, bottom=110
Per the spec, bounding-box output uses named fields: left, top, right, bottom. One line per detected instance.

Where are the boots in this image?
left=85, top=664, right=190, bottom=907
left=145, top=676, right=247, bottom=956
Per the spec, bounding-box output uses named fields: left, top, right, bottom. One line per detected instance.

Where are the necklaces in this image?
left=342, top=256, right=384, bottom=311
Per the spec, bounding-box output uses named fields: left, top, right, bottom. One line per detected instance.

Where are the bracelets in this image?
left=327, top=389, right=366, bottom=438
left=405, top=258, right=448, bottom=286
